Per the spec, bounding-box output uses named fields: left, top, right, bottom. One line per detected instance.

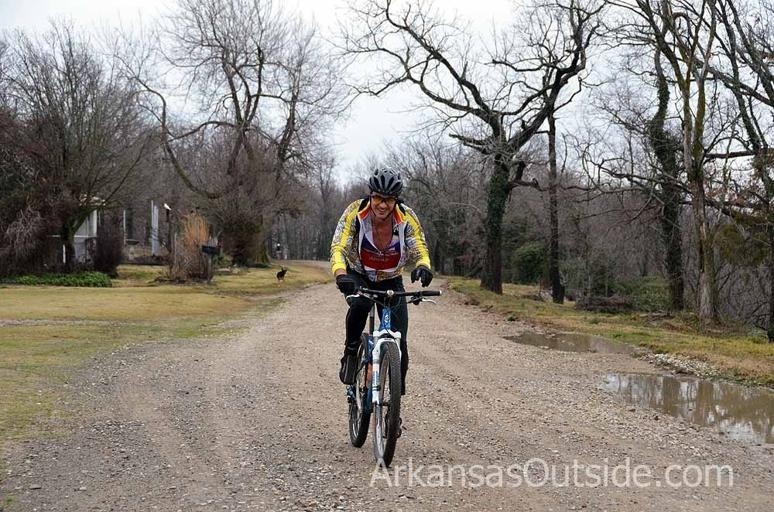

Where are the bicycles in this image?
left=338, top=283, right=442, bottom=468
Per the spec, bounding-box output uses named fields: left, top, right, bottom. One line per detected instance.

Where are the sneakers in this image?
left=340, top=340, right=362, bottom=384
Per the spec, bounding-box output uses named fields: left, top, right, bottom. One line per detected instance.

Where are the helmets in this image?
left=367, top=166, right=403, bottom=197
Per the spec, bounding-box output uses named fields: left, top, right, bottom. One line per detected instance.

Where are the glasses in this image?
left=372, top=195, right=398, bottom=205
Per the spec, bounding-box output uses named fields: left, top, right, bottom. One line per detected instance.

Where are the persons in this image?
left=276, top=242, right=282, bottom=260
left=330, top=166, right=434, bottom=436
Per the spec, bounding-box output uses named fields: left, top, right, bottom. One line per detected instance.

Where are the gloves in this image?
left=336, top=274, right=355, bottom=295
left=411, top=265, right=433, bottom=287
